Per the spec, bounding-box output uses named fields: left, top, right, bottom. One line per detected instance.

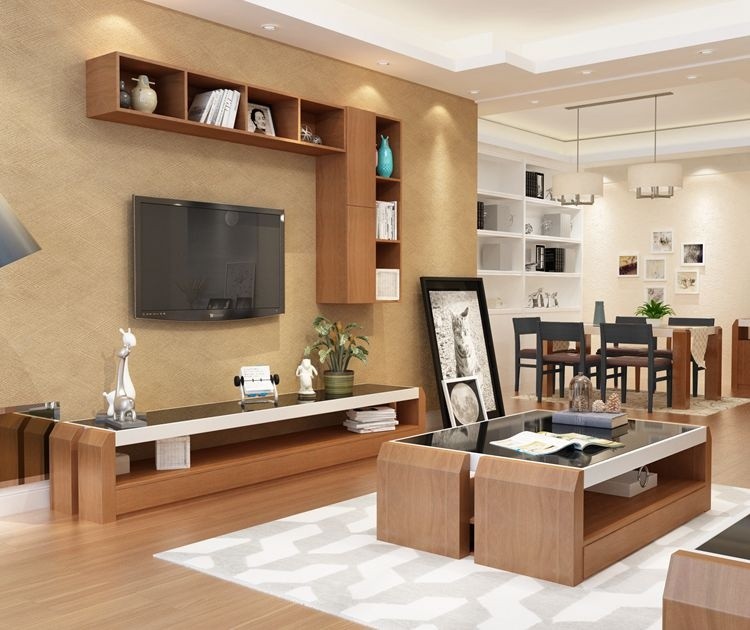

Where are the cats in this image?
left=448, top=306, right=485, bottom=397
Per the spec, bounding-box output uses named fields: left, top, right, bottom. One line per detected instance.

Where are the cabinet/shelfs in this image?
left=85, top=51, right=346, bottom=156
left=476, top=188, right=583, bottom=314
left=315, top=104, right=401, bottom=304
left=49, top=384, right=425, bottom=527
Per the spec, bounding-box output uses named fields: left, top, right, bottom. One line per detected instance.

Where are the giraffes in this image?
left=103, top=326, right=136, bottom=417
left=113, top=344, right=137, bottom=421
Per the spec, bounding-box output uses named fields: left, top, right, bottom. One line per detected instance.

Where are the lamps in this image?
left=553, top=108, right=603, bottom=205
left=627, top=95, right=683, bottom=202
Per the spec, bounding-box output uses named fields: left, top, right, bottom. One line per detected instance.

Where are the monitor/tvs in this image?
left=132, top=193, right=285, bottom=321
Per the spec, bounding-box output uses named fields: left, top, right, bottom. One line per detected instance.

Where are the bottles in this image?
left=593, top=301, right=605, bottom=327
left=377, top=135, right=394, bottom=177
left=130, top=75, right=158, bottom=114
left=120, top=80, right=131, bottom=108
left=568, top=372, right=595, bottom=412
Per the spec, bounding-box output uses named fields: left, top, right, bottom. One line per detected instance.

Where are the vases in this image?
left=377, top=135, right=393, bottom=177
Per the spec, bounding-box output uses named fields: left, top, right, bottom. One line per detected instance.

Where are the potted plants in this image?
left=303, top=315, right=370, bottom=398
left=634, top=300, right=675, bottom=327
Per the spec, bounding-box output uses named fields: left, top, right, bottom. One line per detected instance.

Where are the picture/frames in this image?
left=419, top=275, right=505, bottom=428
left=617, top=229, right=704, bottom=305
left=248, top=103, right=275, bottom=135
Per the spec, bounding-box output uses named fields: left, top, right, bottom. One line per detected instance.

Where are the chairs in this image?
left=513, top=317, right=714, bottom=413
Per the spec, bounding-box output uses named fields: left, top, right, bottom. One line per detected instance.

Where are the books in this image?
left=489, top=431, right=626, bottom=456
left=477, top=201, right=484, bottom=229
left=552, top=410, right=629, bottom=428
left=544, top=248, right=565, bottom=272
left=526, top=170, right=544, bottom=199
left=544, top=214, right=570, bottom=238
left=188, top=89, right=241, bottom=129
left=376, top=201, right=397, bottom=240
left=344, top=407, right=399, bottom=433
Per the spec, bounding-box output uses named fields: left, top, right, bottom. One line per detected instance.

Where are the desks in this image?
left=377, top=409, right=712, bottom=584
left=541, top=325, right=722, bottom=408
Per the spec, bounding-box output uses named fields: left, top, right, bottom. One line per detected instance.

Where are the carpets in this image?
left=515, top=387, right=750, bottom=418
left=154, top=485, right=750, bottom=630
